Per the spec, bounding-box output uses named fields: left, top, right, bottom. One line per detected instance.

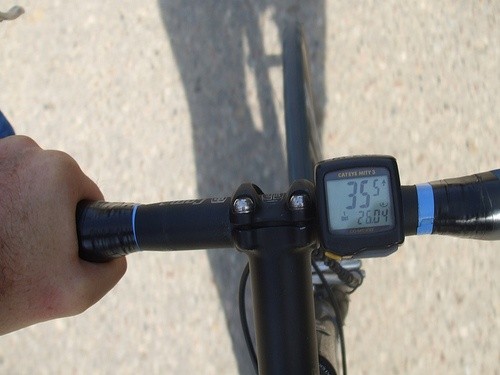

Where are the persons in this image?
left=0, top=106, right=130, bottom=340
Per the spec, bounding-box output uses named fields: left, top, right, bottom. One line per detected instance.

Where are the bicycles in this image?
left=1, top=21, right=500, bottom=375
